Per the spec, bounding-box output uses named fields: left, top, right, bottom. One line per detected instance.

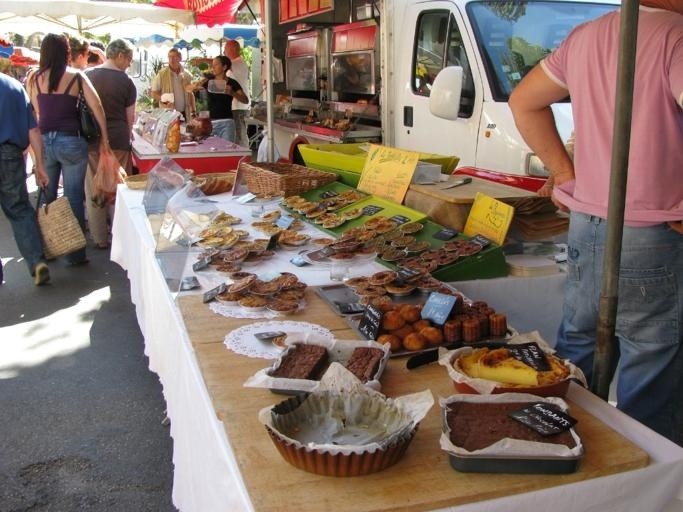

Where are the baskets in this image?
left=241, top=161, right=337, bottom=199
left=192, top=173, right=235, bottom=195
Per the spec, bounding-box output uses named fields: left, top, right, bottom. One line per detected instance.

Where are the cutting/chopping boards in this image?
left=407, top=173, right=538, bottom=205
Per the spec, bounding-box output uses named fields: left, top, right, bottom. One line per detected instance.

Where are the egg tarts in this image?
left=196, top=212, right=275, bottom=274
left=249, top=209, right=335, bottom=246
left=343, top=270, right=455, bottom=307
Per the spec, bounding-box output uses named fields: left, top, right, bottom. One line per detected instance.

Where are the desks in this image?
left=130, top=124, right=252, bottom=175
left=112, top=179, right=682, bottom=512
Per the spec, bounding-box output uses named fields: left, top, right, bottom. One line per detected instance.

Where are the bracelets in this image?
left=191, top=111, right=196, bottom=116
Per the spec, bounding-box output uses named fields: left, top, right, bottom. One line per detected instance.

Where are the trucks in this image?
left=242, top=1, right=628, bottom=182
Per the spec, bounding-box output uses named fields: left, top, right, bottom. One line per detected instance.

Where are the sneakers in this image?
left=34, top=262, right=50, bottom=286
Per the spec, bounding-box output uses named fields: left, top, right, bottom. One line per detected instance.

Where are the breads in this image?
left=283, top=190, right=364, bottom=229
left=371, top=295, right=507, bottom=350
left=444, top=401, right=585, bottom=452
left=331, top=217, right=483, bottom=272
left=271, top=345, right=382, bottom=383
left=214, top=270, right=308, bottom=316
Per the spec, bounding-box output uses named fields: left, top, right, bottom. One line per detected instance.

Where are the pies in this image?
left=449, top=345, right=572, bottom=387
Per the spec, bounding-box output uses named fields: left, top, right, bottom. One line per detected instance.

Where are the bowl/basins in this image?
left=263, top=392, right=417, bottom=479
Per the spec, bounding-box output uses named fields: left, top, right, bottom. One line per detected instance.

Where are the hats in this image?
left=88, top=45, right=106, bottom=63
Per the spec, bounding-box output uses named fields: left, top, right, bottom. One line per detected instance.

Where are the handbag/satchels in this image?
left=33, top=196, right=87, bottom=261
left=77, top=99, right=100, bottom=144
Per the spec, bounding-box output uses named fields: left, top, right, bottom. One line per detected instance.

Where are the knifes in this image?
left=438, top=177, right=472, bottom=191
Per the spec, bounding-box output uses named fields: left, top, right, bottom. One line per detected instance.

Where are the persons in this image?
left=223, top=40, right=251, bottom=148
left=508, top=0, right=683, bottom=446
left=185, top=56, right=249, bottom=143
left=0, top=40, right=50, bottom=286
left=83, top=38, right=137, bottom=250
left=151, top=48, right=196, bottom=123
left=87, top=40, right=105, bottom=68
left=68, top=33, right=107, bottom=70
left=26, top=32, right=109, bottom=264
